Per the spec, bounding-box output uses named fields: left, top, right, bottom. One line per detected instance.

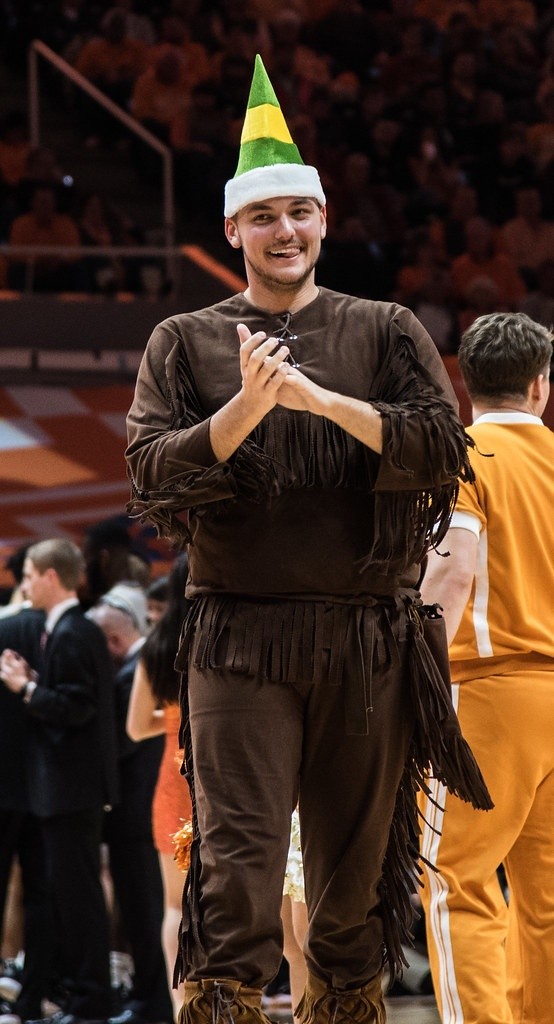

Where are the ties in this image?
left=39, top=632, right=48, bottom=654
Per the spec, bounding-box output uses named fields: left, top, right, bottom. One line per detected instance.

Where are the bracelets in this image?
left=16, top=687, right=26, bottom=700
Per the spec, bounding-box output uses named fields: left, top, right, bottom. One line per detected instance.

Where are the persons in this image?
left=0, top=0, right=554, bottom=1024
left=125, top=55, right=474, bottom=1024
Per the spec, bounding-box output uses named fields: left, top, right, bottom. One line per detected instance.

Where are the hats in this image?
left=222, top=51, right=328, bottom=216
left=99, top=584, right=146, bottom=634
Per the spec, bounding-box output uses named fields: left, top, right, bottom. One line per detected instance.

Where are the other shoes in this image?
left=26, top=1010, right=77, bottom=1024
left=107, top=1010, right=140, bottom=1024
